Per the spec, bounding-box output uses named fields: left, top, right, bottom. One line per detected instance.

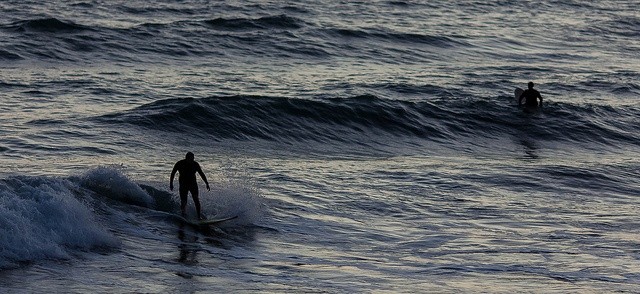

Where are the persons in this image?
left=518, top=82, right=542, bottom=107
left=170, top=152, right=210, bottom=218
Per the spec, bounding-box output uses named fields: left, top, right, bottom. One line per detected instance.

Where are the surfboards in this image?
left=190, top=215, right=238, bottom=224
left=514, top=87, right=547, bottom=119
